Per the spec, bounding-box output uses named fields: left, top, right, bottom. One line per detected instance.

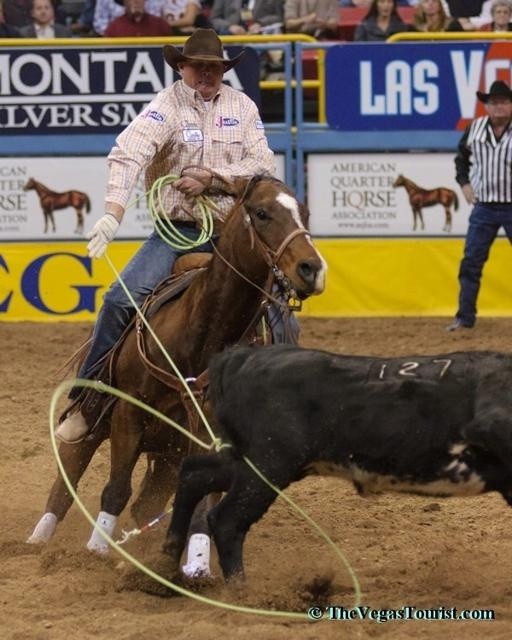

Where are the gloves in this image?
left=85, top=214, right=120, bottom=260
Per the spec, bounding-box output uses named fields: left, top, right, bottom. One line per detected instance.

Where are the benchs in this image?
left=201, top=7, right=421, bottom=101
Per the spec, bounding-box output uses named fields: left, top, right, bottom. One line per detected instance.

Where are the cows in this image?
left=163, top=343, right=512, bottom=588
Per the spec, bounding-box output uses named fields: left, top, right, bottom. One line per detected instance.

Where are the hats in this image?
left=476, top=81, right=512, bottom=102
left=162, top=28, right=247, bottom=74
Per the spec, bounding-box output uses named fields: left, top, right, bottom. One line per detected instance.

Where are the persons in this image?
left=446, top=79, right=512, bottom=330
left=52, top=28, right=299, bottom=445
left=1, top=0, right=511, bottom=106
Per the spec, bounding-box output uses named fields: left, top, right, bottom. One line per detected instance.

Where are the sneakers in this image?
left=446, top=323, right=472, bottom=332
left=53, top=408, right=88, bottom=445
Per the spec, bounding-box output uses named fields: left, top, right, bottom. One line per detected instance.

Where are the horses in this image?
left=22, top=178, right=91, bottom=234
left=23, top=172, right=329, bottom=580
left=392, top=174, right=458, bottom=232
